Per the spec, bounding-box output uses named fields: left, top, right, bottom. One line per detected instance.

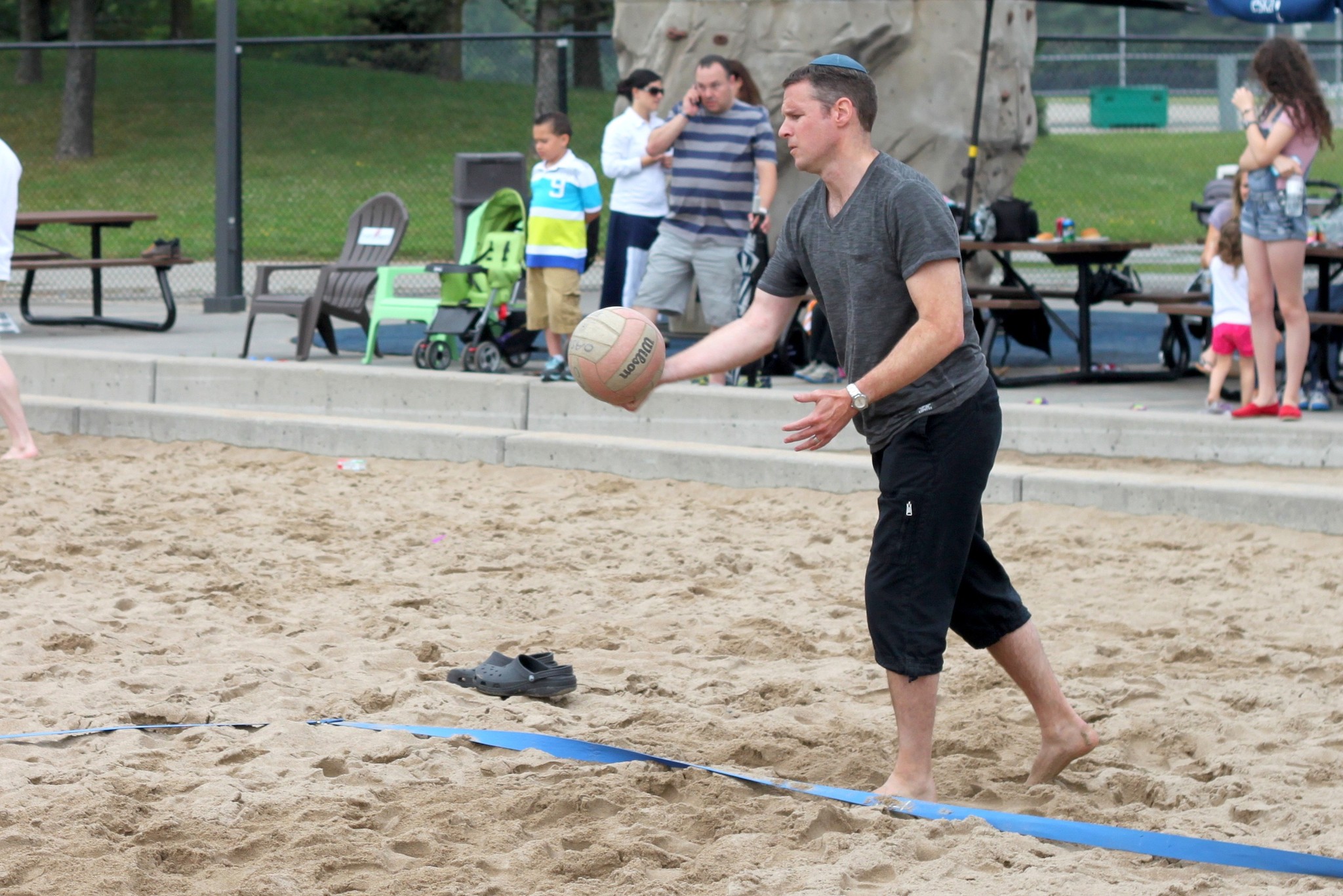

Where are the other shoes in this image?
left=1298, top=387, right=1308, bottom=409
left=1205, top=396, right=1230, bottom=413
left=1308, top=391, right=1333, bottom=410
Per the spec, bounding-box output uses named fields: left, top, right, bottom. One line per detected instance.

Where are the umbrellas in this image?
left=725, top=210, right=760, bottom=388
left=744, top=207, right=770, bottom=388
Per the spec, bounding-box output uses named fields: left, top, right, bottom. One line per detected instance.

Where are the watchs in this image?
left=846, top=383, right=868, bottom=411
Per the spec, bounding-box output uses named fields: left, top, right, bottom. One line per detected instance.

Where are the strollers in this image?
left=412, top=188, right=540, bottom=372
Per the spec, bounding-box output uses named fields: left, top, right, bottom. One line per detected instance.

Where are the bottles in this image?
left=1284, top=174, right=1304, bottom=217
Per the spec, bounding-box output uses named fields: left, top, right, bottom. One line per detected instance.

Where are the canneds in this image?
left=1056, top=216, right=1075, bottom=243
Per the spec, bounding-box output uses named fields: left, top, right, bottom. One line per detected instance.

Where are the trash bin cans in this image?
left=1088, top=83, right=1168, bottom=127
left=451, top=151, right=526, bottom=264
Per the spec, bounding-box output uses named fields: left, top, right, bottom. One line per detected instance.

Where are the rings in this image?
left=812, top=436, right=820, bottom=443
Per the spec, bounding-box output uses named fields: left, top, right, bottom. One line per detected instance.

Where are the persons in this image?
left=623, top=52, right=1098, bottom=805
left=1205, top=217, right=1255, bottom=415
left=1231, top=36, right=1337, bottom=422
left=600, top=54, right=843, bottom=387
left=1195, top=167, right=1250, bottom=373
left=0, top=140, right=37, bottom=460
left=525, top=111, right=603, bottom=381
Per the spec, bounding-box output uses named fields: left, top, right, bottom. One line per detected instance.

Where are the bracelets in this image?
left=679, top=111, right=693, bottom=121
left=1241, top=108, right=1254, bottom=115
left=1241, top=120, right=1258, bottom=130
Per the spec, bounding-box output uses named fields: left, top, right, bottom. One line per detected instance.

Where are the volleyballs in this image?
left=568, top=306, right=666, bottom=406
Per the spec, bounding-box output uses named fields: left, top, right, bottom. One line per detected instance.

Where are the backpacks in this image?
left=761, top=298, right=811, bottom=375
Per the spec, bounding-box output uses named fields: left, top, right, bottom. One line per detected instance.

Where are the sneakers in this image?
left=541, top=354, right=566, bottom=380
left=561, top=365, right=574, bottom=381
left=805, top=362, right=841, bottom=383
left=794, top=360, right=818, bottom=378
left=1231, top=402, right=1280, bottom=418
left=1278, top=404, right=1301, bottom=421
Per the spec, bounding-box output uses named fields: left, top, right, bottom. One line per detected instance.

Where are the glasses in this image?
left=644, top=86, right=664, bottom=97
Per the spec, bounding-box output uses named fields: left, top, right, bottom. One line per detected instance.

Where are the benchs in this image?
left=0, top=250, right=194, bottom=331
left=944, top=285, right=1343, bottom=396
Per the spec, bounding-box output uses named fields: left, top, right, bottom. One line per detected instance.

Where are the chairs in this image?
left=238, top=194, right=409, bottom=364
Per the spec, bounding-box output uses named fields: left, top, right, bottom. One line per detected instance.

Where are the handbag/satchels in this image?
left=968, top=197, right=1039, bottom=241
left=1074, top=259, right=1143, bottom=306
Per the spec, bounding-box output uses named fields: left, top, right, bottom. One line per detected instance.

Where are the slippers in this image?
left=446, top=651, right=577, bottom=697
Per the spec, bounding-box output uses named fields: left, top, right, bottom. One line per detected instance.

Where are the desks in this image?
left=958, top=240, right=1151, bottom=385
left=8, top=210, right=159, bottom=317
left=1305, top=245, right=1343, bottom=394
